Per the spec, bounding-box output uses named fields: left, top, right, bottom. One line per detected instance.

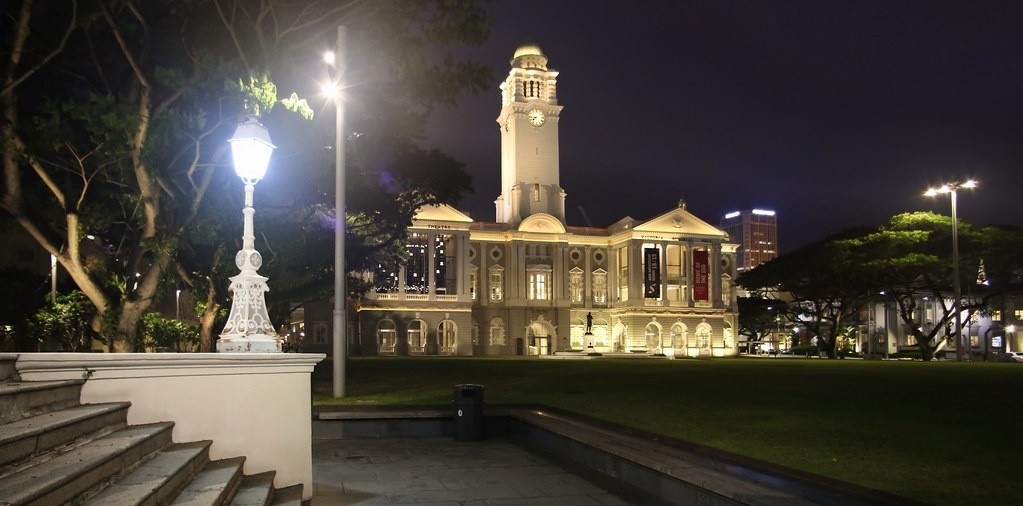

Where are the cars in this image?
left=1006, top=352, right=1023, bottom=363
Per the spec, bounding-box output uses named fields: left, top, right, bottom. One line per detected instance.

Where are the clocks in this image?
left=527, top=107, right=546, bottom=128
left=505, top=114, right=512, bottom=132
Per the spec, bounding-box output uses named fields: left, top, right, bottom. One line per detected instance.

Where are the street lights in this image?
left=880, top=290, right=889, bottom=360
left=214, top=116, right=284, bottom=354
left=968, top=279, right=990, bottom=361
left=315, top=27, right=351, bottom=398
left=922, top=296, right=928, bottom=335
left=921, top=178, right=979, bottom=363
left=50, top=234, right=96, bottom=304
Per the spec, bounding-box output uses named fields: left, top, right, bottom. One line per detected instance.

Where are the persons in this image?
left=586, top=312, right=593, bottom=333
